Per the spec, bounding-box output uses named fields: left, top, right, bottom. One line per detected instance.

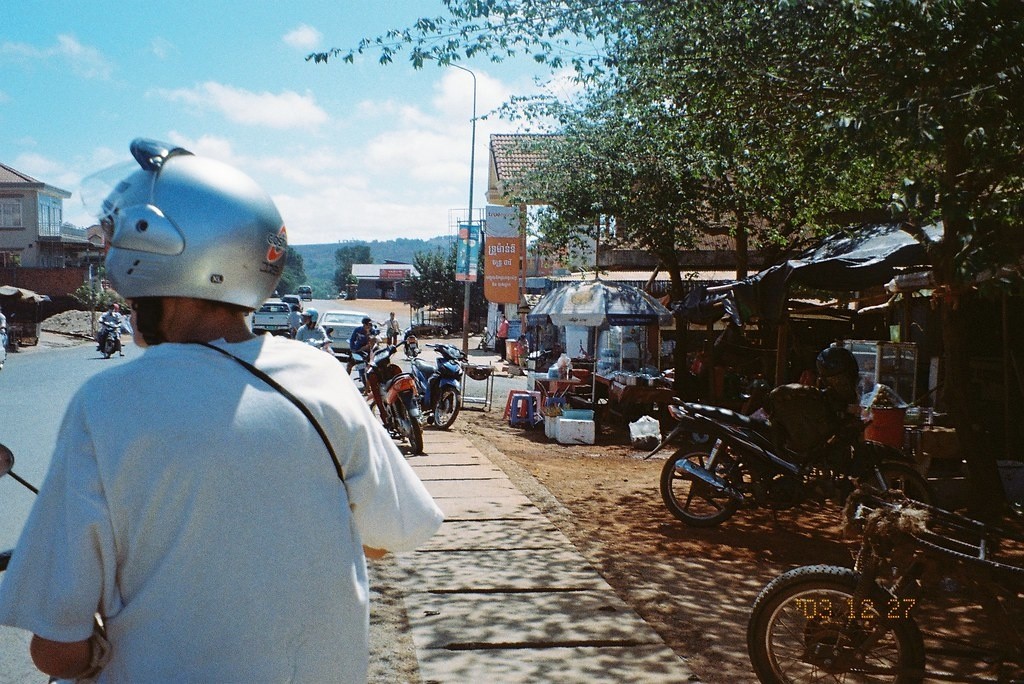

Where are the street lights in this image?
left=422, top=51, right=476, bottom=357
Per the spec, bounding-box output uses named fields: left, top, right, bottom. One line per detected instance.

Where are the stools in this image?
left=527, top=391, right=541, bottom=415
left=502, top=390, right=527, bottom=418
left=511, top=393, right=534, bottom=428
left=545, top=397, right=565, bottom=409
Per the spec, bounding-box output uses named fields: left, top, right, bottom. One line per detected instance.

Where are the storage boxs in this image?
left=544, top=415, right=556, bottom=438
left=555, top=416, right=596, bottom=444
left=561, top=409, right=594, bottom=419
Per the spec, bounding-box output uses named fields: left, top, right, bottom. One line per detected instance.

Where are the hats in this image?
left=362, top=317, right=374, bottom=324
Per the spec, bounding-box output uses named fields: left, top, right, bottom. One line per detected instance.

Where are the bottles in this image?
left=862, top=406, right=870, bottom=422
left=596, top=347, right=664, bottom=380
left=568, top=366, right=573, bottom=380
left=548, top=366, right=566, bottom=380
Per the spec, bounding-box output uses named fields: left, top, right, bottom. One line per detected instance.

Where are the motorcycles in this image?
left=95, top=321, right=125, bottom=359
left=0, top=441, right=43, bottom=571
left=659, top=345, right=1024, bottom=684
left=353, top=328, right=469, bottom=455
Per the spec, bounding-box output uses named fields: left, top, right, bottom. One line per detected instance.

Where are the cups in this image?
left=889, top=325, right=901, bottom=343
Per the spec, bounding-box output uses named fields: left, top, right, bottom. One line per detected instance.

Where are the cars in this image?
left=298, top=285, right=313, bottom=301
left=251, top=293, right=303, bottom=339
left=316, top=312, right=378, bottom=354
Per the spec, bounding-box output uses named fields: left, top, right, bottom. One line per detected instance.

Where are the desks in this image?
left=528, top=373, right=581, bottom=429
left=604, top=381, right=672, bottom=428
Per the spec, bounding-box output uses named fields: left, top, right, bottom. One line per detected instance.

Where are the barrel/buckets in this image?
left=859, top=401, right=909, bottom=452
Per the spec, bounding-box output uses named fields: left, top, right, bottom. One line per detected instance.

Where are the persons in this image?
left=286, top=305, right=304, bottom=339
left=346, top=318, right=378, bottom=376
left=0, top=306, right=5, bottom=335
left=517, top=334, right=529, bottom=366
left=294, top=306, right=331, bottom=352
left=0, top=138, right=448, bottom=684
left=498, top=314, right=512, bottom=362
left=377, top=311, right=401, bottom=353
left=96, top=303, right=124, bottom=355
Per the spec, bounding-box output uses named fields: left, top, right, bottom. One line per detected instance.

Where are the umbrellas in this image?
left=526, top=277, right=674, bottom=403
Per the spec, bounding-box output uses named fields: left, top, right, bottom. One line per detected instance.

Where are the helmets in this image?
left=101, top=153, right=287, bottom=312
left=816, top=347, right=859, bottom=383
left=302, top=309, right=318, bottom=323
left=111, top=304, right=119, bottom=311
